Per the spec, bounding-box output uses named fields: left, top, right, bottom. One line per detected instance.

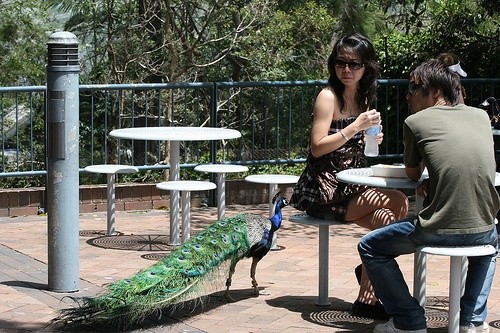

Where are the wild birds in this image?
left=37, top=189, right=290, bottom=333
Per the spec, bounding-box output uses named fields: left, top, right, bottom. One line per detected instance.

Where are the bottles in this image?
left=364, top=109, right=379, bottom=157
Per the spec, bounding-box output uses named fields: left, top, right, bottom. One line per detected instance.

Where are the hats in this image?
left=449, top=60, right=468, bottom=78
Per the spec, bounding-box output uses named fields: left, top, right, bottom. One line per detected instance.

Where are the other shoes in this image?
left=355, top=263, right=363, bottom=286
left=352, top=298, right=388, bottom=320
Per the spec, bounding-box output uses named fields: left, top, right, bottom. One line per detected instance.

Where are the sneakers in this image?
left=373, top=316, right=427, bottom=333
left=459, top=322, right=492, bottom=333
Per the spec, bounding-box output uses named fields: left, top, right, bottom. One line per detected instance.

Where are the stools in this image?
left=85, top=164, right=139, bottom=235
left=194, top=164, right=249, bottom=220
left=416, top=244, right=496, bottom=333
left=288, top=215, right=344, bottom=306
left=156, top=180, right=218, bottom=243
left=244, top=174, right=301, bottom=219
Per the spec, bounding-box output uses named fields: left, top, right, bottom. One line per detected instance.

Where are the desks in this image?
left=109, top=127, right=242, bottom=245
left=336, top=168, right=500, bottom=307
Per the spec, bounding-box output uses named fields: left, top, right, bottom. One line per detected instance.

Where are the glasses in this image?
left=408, top=82, right=423, bottom=94
left=333, top=59, right=364, bottom=70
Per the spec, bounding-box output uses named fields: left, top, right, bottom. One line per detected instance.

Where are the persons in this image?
left=358, top=54, right=500, bottom=333
left=288, top=33, right=410, bottom=320
